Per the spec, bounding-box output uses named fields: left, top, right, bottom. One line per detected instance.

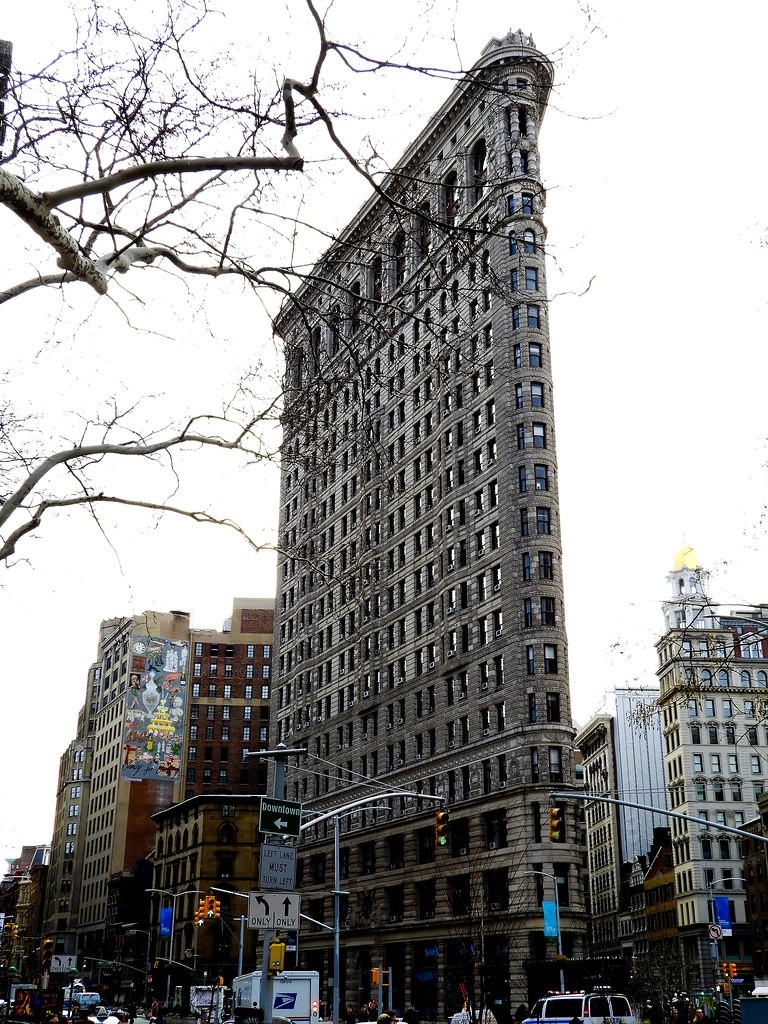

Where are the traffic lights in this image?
left=206, top=895, right=215, bottom=917
left=549, top=807, right=562, bottom=842
left=4, top=960, right=8, bottom=968
left=42, top=937, right=54, bottom=969
left=729, top=963, right=737, bottom=977
left=154, top=961, right=159, bottom=969
left=198, top=918, right=205, bottom=927
left=13, top=924, right=20, bottom=938
left=4, top=924, right=11, bottom=941
left=720, top=983, right=729, bottom=992
left=194, top=911, right=199, bottom=926
left=215, top=900, right=221, bottom=918
left=722, top=963, right=730, bottom=979
left=199, top=900, right=206, bottom=918
left=435, top=811, right=450, bottom=849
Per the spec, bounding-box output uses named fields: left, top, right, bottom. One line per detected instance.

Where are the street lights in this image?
left=522, top=869, right=565, bottom=993
left=128, top=929, right=151, bottom=1010
left=145, top=887, right=205, bottom=1006
left=709, top=878, right=747, bottom=1002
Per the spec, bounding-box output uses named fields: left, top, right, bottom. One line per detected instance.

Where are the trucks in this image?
left=10, top=983, right=38, bottom=1009
left=173, top=970, right=319, bottom=1024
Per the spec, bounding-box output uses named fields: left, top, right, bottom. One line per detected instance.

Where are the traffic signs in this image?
left=49, top=955, right=77, bottom=972
left=258, top=796, right=302, bottom=838
left=247, top=890, right=301, bottom=931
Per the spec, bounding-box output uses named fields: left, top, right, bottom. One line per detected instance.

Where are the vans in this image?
left=521, top=984, right=636, bottom=1024
left=61, top=985, right=122, bottom=1024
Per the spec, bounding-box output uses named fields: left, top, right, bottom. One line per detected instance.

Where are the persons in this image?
left=320, top=998, right=422, bottom=1024
left=631, top=1002, right=715, bottom=1024
left=41, top=995, right=168, bottom=1024
left=24, top=991, right=35, bottom=1016
